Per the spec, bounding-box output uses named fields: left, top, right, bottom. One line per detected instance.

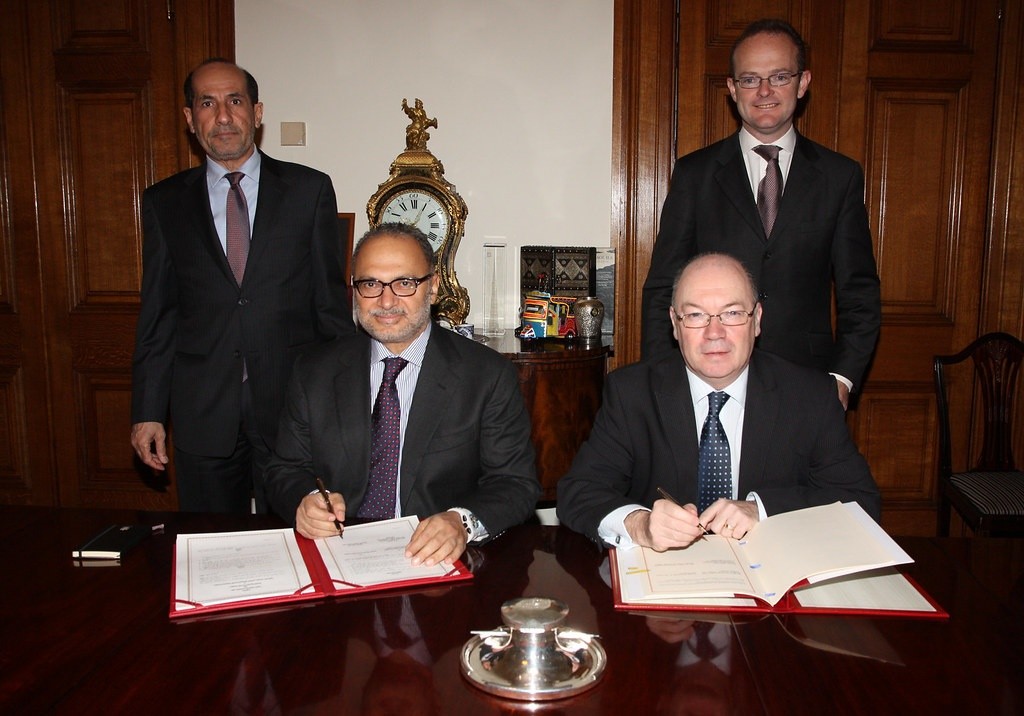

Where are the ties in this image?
left=376, top=596, right=410, bottom=650
left=698, top=392, right=733, bottom=535
left=750, top=144, right=784, bottom=237
left=356, top=356, right=408, bottom=519
left=693, top=619, right=721, bottom=660
left=223, top=171, right=251, bottom=382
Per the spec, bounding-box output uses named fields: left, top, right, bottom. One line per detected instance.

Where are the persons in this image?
left=265, top=223, right=543, bottom=563
left=641, top=19, right=883, bottom=422
left=130, top=59, right=346, bottom=513
left=556, top=252, right=883, bottom=552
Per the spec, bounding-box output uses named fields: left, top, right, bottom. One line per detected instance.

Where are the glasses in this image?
left=733, top=70, right=806, bottom=89
left=352, top=274, right=433, bottom=298
left=672, top=300, right=757, bottom=329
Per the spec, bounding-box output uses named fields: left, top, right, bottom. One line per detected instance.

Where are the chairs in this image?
left=932, top=330, right=1024, bottom=578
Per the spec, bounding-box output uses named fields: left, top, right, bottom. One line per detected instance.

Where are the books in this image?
left=609, top=501, right=950, bottom=619
left=169, top=514, right=473, bottom=620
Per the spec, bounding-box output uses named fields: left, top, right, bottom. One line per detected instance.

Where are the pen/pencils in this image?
left=315, top=478, right=344, bottom=538
left=656, top=485, right=708, bottom=534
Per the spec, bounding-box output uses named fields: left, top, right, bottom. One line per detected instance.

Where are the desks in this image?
left=473, top=328, right=610, bottom=505
left=0, top=505, right=1024, bottom=716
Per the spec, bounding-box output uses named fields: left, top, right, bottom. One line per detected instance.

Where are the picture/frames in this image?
left=337, top=212, right=355, bottom=320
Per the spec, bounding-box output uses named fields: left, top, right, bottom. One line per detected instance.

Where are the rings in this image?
left=723, top=523, right=734, bottom=530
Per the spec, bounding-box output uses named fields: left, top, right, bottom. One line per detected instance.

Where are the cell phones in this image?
left=72, top=523, right=152, bottom=559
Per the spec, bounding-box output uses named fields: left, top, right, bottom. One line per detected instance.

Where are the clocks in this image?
left=366, top=98, right=470, bottom=329
